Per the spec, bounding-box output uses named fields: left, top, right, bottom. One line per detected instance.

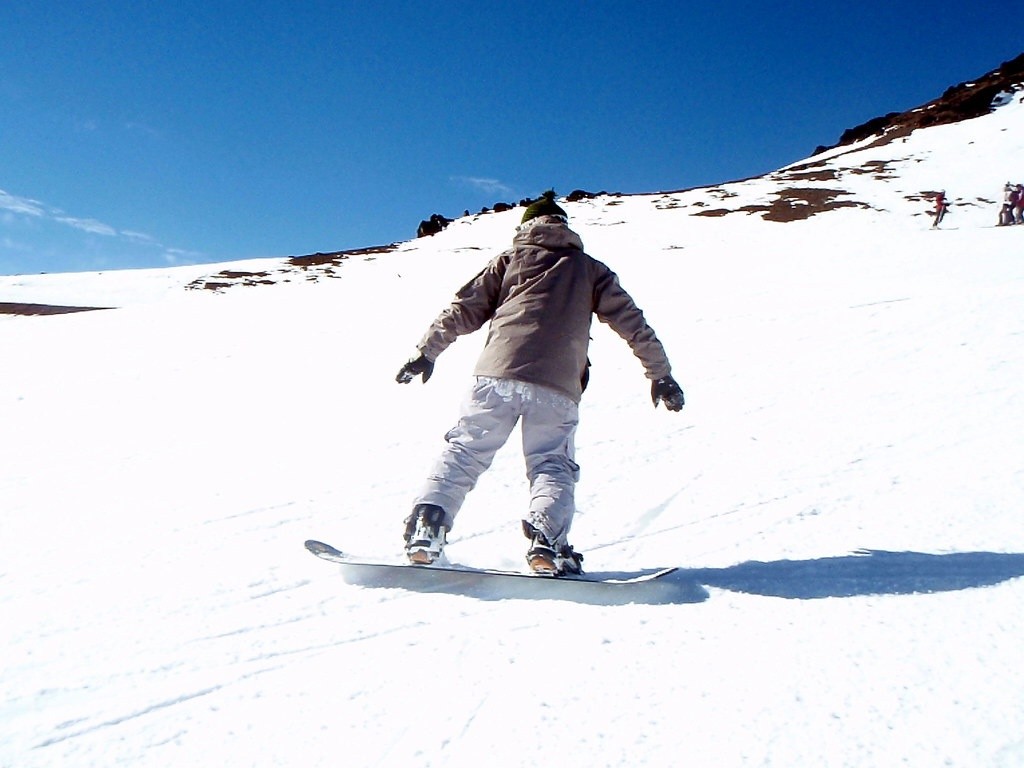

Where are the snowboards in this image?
left=302, top=538, right=681, bottom=585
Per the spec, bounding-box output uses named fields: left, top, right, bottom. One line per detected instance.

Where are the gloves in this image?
left=651, top=376, right=685, bottom=413
left=396, top=345, right=435, bottom=385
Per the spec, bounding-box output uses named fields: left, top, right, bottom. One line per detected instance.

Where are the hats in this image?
left=521, top=198, right=568, bottom=224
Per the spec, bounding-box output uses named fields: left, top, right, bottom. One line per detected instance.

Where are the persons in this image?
left=396, top=195, right=684, bottom=572
left=998, top=181, right=1024, bottom=225
left=933, top=189, right=947, bottom=226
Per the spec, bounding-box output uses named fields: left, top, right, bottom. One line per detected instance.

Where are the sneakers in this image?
left=402, top=504, right=449, bottom=564
left=527, top=532, right=579, bottom=570
